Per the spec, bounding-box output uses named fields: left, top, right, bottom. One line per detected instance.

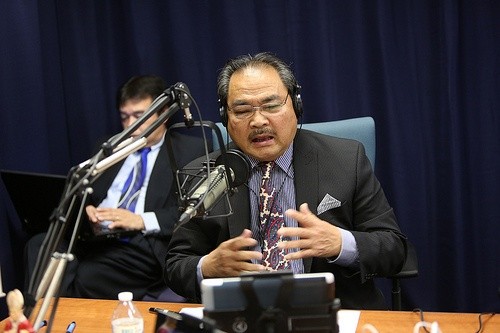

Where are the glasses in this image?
left=226, top=88, right=289, bottom=120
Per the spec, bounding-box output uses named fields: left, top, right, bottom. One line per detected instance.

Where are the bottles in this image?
left=111, top=292, right=144, bottom=333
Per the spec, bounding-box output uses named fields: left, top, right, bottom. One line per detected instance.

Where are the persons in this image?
left=26, top=71, right=211, bottom=299
left=166, top=52, right=408, bottom=310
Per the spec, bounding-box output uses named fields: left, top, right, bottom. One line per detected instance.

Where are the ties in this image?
left=116, top=146, right=151, bottom=242
left=255, top=160, right=291, bottom=272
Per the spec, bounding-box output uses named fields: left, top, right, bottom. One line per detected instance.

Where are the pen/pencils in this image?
left=40, top=320, right=48, bottom=326
left=65, top=320, right=76, bottom=333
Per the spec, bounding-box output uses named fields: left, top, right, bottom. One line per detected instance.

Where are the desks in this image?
left=27, top=297, right=500, bottom=333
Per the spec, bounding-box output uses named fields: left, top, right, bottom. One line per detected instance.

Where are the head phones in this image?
left=218, top=84, right=304, bottom=126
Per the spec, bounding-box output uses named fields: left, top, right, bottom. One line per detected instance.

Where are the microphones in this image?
left=178, top=149, right=253, bottom=225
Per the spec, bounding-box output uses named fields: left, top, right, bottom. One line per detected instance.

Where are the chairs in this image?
left=210, top=117, right=417, bottom=311
